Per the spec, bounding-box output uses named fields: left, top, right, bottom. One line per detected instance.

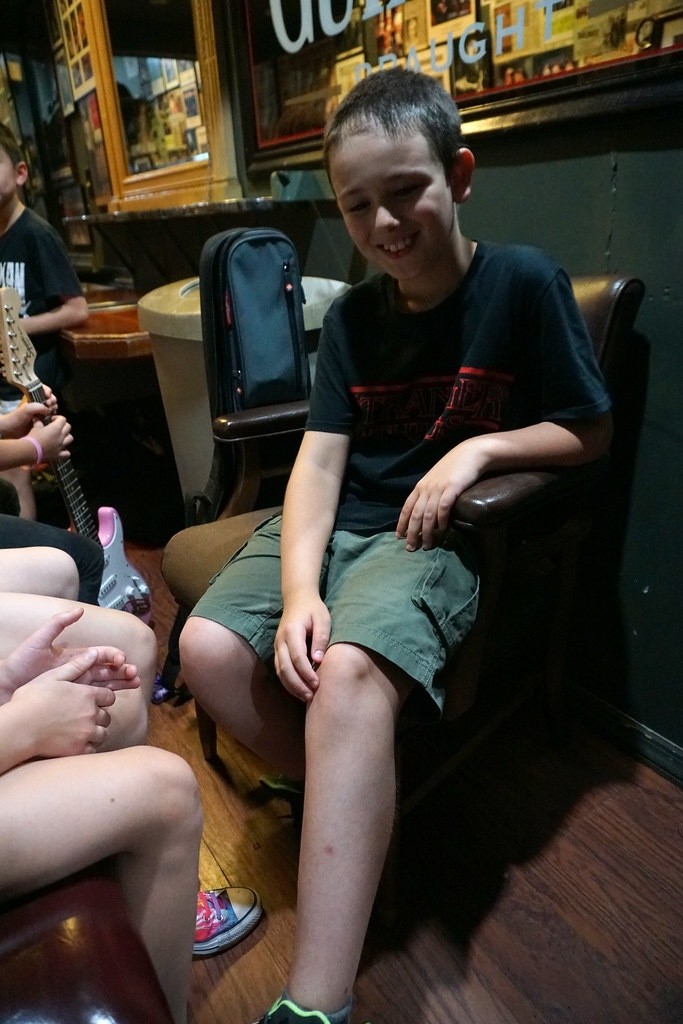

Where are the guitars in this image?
left=0, top=282, right=156, bottom=633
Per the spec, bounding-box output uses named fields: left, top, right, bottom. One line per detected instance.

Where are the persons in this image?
left=0, top=382, right=105, bottom=607
left=0, top=124, right=93, bottom=529
left=179, top=68, right=613, bottom=1024
left=0, top=608, right=263, bottom=1024
left=0, top=547, right=158, bottom=709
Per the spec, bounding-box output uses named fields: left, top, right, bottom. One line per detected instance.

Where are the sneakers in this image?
left=192, top=885, right=262, bottom=953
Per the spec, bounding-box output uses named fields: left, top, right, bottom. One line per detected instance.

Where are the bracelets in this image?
left=21, top=436, right=42, bottom=470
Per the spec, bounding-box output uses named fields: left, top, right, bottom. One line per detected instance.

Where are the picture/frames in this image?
left=224, top=0, right=683, bottom=180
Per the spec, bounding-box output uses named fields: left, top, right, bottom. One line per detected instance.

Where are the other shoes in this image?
left=256, top=773, right=303, bottom=841
left=249, top=994, right=353, bottom=1024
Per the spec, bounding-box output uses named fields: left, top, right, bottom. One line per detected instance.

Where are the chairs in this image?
left=197, top=275, right=649, bottom=831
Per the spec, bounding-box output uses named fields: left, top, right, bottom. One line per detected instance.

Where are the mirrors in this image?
left=76, top=0, right=237, bottom=213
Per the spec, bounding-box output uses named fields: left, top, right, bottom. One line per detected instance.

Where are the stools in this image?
left=1, top=864, right=179, bottom=1023
